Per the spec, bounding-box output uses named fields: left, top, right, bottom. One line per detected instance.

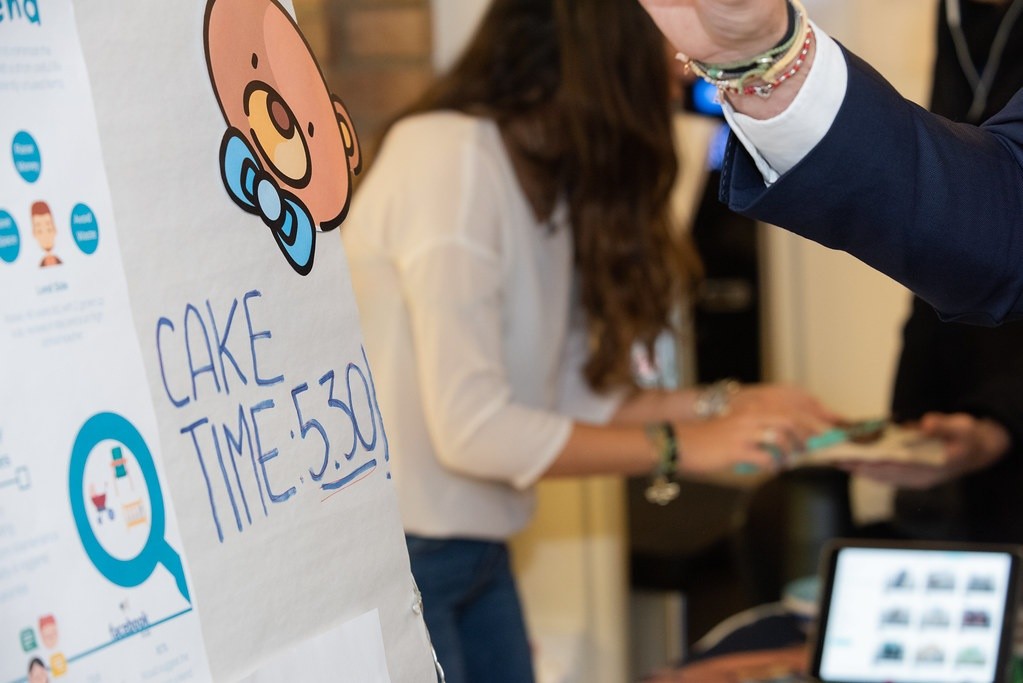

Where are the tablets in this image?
left=810, top=537, right=1023, bottom=683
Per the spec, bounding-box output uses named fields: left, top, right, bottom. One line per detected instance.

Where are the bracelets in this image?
left=674, top=0, right=813, bottom=100
left=644, top=418, right=680, bottom=505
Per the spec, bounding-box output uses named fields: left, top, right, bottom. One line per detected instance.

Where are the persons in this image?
left=342, top=0, right=841, bottom=681
left=638, top=0, right=1023, bottom=324
left=872, top=0, right=1023, bottom=614
